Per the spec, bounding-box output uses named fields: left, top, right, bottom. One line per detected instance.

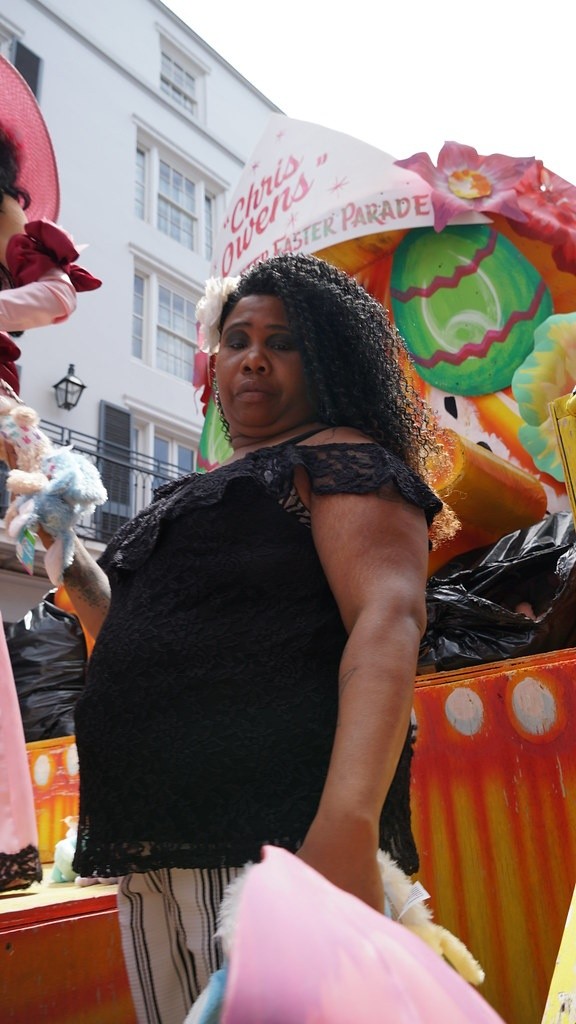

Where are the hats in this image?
left=1, top=54, right=62, bottom=224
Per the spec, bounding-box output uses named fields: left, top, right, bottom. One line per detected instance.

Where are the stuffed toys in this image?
left=9, top=444, right=108, bottom=588
left=213, top=844, right=486, bottom=985
left=0, top=395, right=52, bottom=528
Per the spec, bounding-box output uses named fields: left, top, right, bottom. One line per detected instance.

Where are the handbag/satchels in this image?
left=415, top=509, right=576, bottom=674
left=3, top=597, right=88, bottom=744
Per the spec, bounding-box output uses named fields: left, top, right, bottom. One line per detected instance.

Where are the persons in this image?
left=19, top=250, right=460, bottom=1023
left=0, top=128, right=77, bottom=898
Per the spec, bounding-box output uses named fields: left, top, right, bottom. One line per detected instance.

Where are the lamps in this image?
left=52, top=363, right=89, bottom=412
